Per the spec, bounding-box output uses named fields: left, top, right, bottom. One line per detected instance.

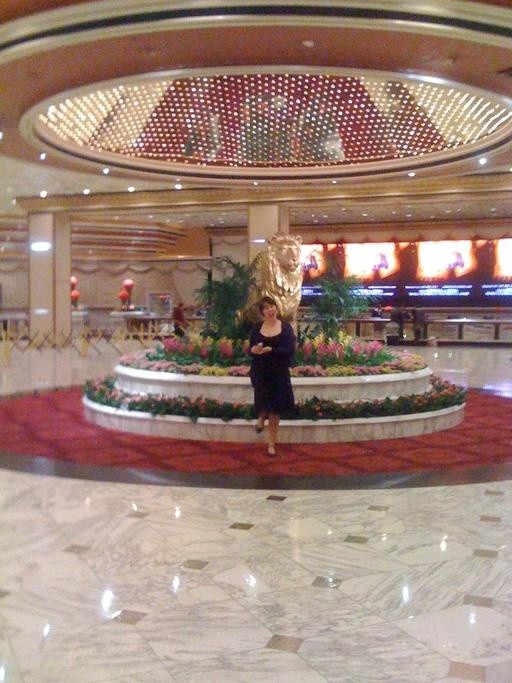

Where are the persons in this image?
left=173, top=301, right=187, bottom=336
left=371, top=302, right=384, bottom=316
left=388, top=304, right=405, bottom=340
left=411, top=307, right=424, bottom=344
left=247, top=294, right=296, bottom=457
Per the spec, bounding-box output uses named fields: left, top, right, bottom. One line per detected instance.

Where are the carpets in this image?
left=1, top=383, right=512, bottom=476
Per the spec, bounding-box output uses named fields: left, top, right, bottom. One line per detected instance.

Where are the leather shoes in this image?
left=254, top=423, right=277, bottom=458
